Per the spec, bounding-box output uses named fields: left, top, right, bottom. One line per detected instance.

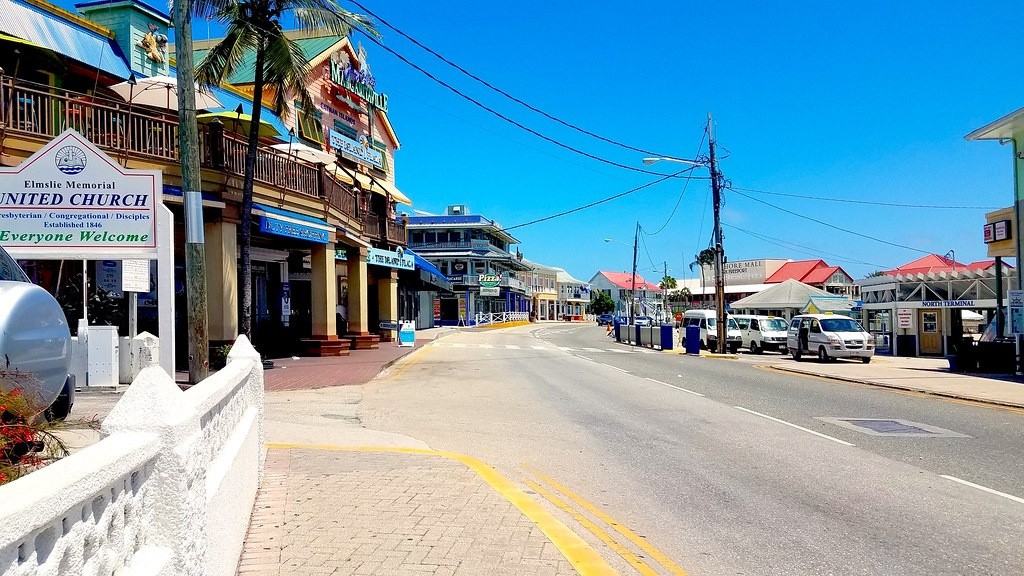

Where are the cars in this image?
left=646, top=316, right=665, bottom=326
left=634, top=316, right=650, bottom=327
left=599, top=314, right=614, bottom=326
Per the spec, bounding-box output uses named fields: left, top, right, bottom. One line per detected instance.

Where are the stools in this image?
left=13, top=95, right=39, bottom=133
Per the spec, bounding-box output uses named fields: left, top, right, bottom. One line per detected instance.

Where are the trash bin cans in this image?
left=686, top=325, right=700, bottom=354
left=615, top=321, right=626, bottom=342
left=661, top=324, right=673, bottom=350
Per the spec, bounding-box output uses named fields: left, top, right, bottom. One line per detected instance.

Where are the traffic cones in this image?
left=607, top=323, right=610, bottom=331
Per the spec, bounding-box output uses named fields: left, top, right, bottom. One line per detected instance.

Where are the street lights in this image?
left=604, top=239, right=637, bottom=326
left=641, top=156, right=725, bottom=352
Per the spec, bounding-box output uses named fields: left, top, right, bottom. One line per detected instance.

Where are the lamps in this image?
left=327, top=148, right=342, bottom=219
left=227, top=103, right=243, bottom=180
left=366, top=179, right=374, bottom=222
left=124, top=72, right=138, bottom=168
left=945, top=249, right=955, bottom=271
left=281, top=127, right=295, bottom=206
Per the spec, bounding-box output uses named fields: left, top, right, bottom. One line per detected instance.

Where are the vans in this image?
left=728, top=315, right=790, bottom=355
left=787, top=312, right=875, bottom=364
left=679, top=309, right=742, bottom=354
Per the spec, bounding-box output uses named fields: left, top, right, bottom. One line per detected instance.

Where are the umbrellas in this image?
left=264, top=140, right=338, bottom=191
left=193, top=110, right=283, bottom=177
left=106, top=76, right=226, bottom=112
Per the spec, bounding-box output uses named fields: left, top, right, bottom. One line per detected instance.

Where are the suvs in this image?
left=0, top=245, right=76, bottom=465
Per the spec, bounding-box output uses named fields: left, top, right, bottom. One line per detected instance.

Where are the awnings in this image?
left=325, top=162, right=412, bottom=205
left=403, top=247, right=452, bottom=286
